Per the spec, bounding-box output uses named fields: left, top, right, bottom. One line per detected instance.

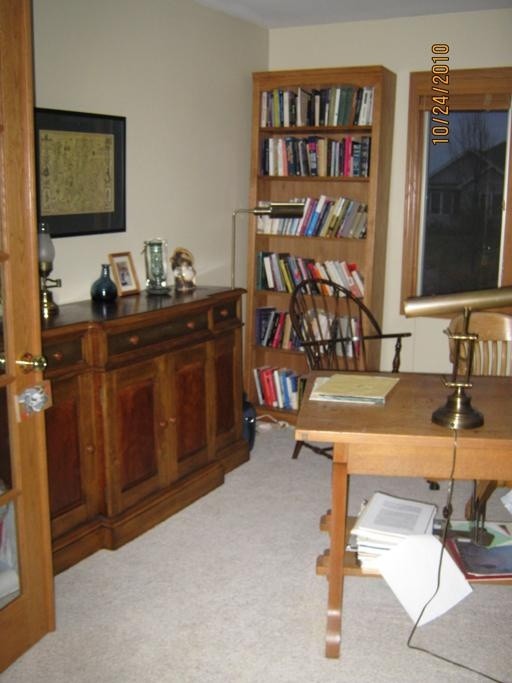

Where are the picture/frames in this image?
left=36, top=107, right=126, bottom=237
left=110, top=252, right=141, bottom=296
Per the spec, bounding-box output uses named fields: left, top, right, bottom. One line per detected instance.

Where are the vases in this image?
left=90, top=264, right=118, bottom=301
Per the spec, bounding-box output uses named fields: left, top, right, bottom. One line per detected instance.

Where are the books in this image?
left=251, top=366, right=308, bottom=413
left=309, top=372, right=401, bottom=406
left=256, top=251, right=364, bottom=299
left=345, top=491, right=512, bottom=628
left=260, top=84, right=374, bottom=129
left=254, top=306, right=360, bottom=360
left=256, top=194, right=368, bottom=239
left=260, top=134, right=371, bottom=178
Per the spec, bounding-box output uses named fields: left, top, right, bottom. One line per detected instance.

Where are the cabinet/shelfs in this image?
left=214, top=295, right=251, bottom=467
left=101, top=309, right=217, bottom=551
left=245, top=65, right=398, bottom=424
left=42, top=326, right=97, bottom=569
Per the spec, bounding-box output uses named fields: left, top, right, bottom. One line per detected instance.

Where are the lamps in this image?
left=38, top=222, right=62, bottom=318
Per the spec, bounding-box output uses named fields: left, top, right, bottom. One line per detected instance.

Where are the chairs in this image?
left=290, top=276, right=412, bottom=458
left=449, top=314, right=512, bottom=520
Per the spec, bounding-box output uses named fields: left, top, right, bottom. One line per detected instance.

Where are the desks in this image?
left=295, top=370, right=512, bottom=661
